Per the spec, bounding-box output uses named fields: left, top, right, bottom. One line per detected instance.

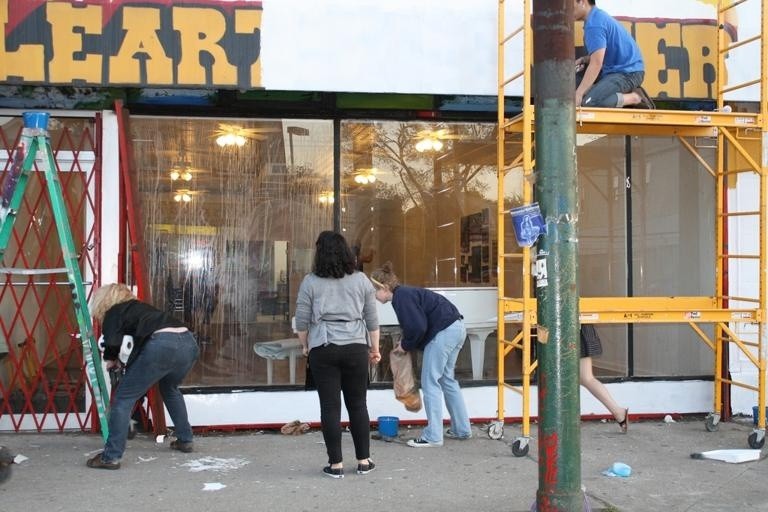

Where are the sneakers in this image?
left=87, top=453, right=121, bottom=470
left=170, top=440, right=194, bottom=453
left=322, top=464, right=345, bottom=479
left=633, top=87, right=656, bottom=110
left=444, top=428, right=471, bottom=439
left=356, top=458, right=376, bottom=475
left=407, top=436, right=440, bottom=448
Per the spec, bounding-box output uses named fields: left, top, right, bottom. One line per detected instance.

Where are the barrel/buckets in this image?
left=753, top=406, right=768, bottom=425
left=23, top=111, right=49, bottom=129
left=378, top=416, right=399, bottom=437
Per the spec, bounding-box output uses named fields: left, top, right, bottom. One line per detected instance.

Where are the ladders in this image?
left=0, top=126, right=110, bottom=456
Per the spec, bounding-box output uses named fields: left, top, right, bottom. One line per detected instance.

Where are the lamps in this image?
left=216, top=134, right=247, bottom=148
left=352, top=170, right=378, bottom=187
left=171, top=165, right=193, bottom=182
left=416, top=136, right=443, bottom=152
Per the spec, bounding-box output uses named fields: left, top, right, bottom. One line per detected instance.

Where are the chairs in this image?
left=254, top=317, right=304, bottom=385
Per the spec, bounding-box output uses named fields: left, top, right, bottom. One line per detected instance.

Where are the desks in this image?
left=368, top=286, right=498, bottom=380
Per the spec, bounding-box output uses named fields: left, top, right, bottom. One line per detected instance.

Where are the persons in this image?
left=86, top=283, right=200, bottom=471
left=296, top=230, right=382, bottom=480
left=369, top=260, right=472, bottom=449
left=578, top=324, right=629, bottom=435
left=573, top=0, right=657, bottom=110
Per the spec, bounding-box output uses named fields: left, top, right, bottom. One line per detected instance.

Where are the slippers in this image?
left=618, top=407, right=629, bottom=432
